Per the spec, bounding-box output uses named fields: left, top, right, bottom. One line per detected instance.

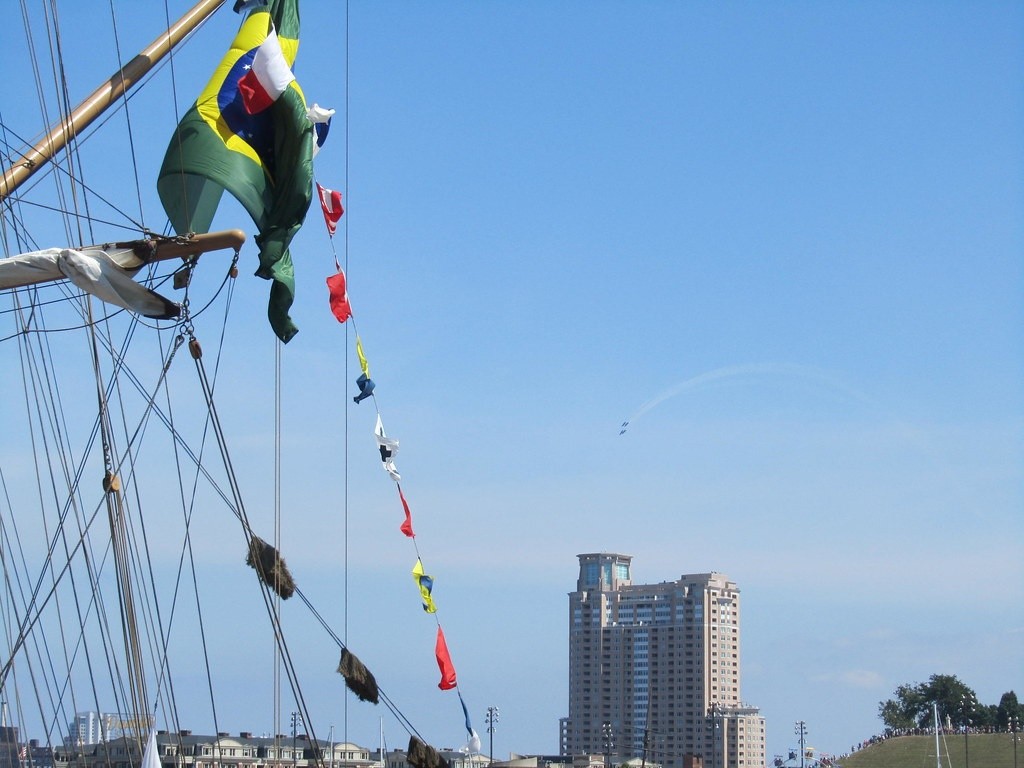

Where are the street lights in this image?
left=1008, top=717, right=1020, bottom=768
left=290, top=711, right=302, bottom=768
left=485, top=706, right=500, bottom=766
left=603, top=724, right=614, bottom=768
left=957, top=693, right=976, bottom=768
left=795, top=720, right=808, bottom=768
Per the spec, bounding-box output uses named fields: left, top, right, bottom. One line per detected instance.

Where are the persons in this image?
left=818, top=724, right=1008, bottom=763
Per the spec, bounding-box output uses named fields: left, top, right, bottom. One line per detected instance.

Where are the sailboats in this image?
left=0, top=1, right=482, bottom=768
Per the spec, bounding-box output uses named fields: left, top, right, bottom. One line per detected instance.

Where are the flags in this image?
left=154, top=0, right=484, bottom=758
